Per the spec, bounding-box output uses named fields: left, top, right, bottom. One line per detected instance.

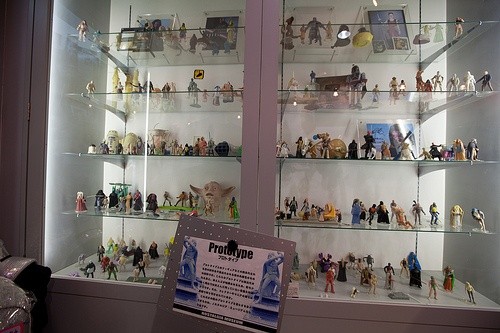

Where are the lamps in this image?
left=338, top=22, right=353, bottom=41
left=412, top=34, right=432, bottom=45
left=352, top=26, right=375, bottom=50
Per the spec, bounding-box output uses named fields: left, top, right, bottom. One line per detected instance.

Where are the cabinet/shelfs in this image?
left=26, top=0, right=500, bottom=333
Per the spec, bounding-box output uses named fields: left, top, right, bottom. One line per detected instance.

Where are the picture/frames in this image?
left=199, top=10, right=243, bottom=58
left=355, top=117, right=421, bottom=162
left=129, top=12, right=178, bottom=60
left=360, top=3, right=419, bottom=56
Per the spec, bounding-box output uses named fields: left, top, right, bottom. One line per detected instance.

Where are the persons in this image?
left=152, top=20, right=161, bottom=31
left=78, top=236, right=170, bottom=285
left=386, top=11, right=402, bottom=38
left=180, top=236, right=199, bottom=290
left=212, top=38, right=232, bottom=57
left=98, top=136, right=215, bottom=156
left=374, top=136, right=486, bottom=304
left=187, top=78, right=232, bottom=105
left=189, top=33, right=198, bottom=52
left=389, top=17, right=494, bottom=105
left=387, top=124, right=404, bottom=157
left=281, top=16, right=381, bottom=112
left=86, top=80, right=96, bottom=98
left=77, top=19, right=102, bottom=53
left=116, top=80, right=176, bottom=101
left=276, top=118, right=377, bottom=298
left=75, top=181, right=240, bottom=219
left=251, top=248, right=283, bottom=304
left=179, top=22, right=188, bottom=43
left=227, top=18, right=235, bottom=42
